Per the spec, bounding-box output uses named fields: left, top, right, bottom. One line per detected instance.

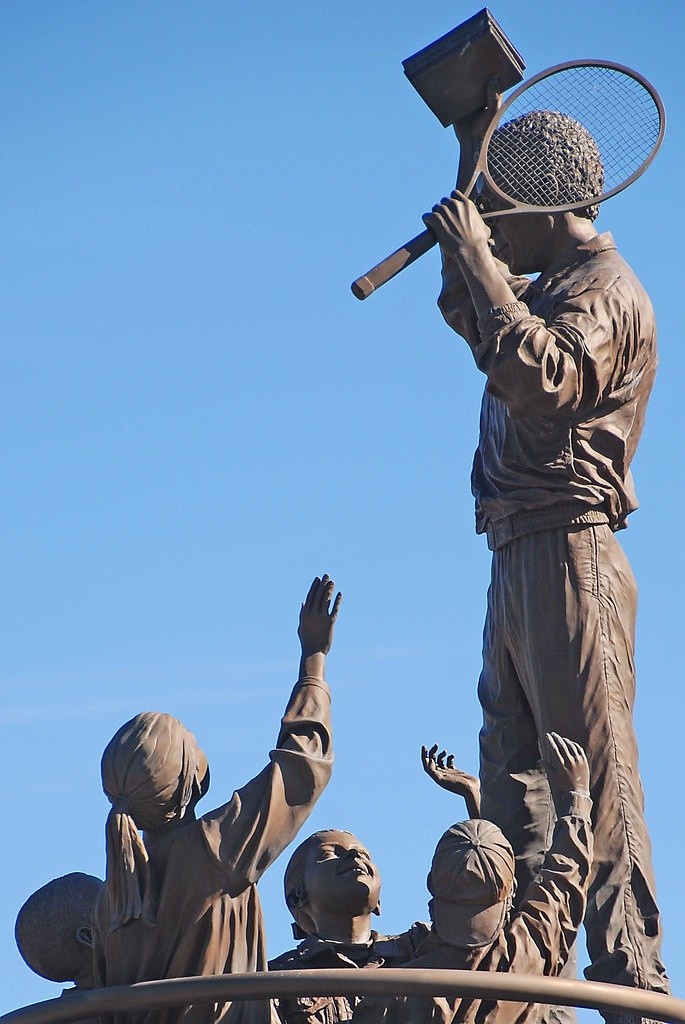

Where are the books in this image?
left=402, top=6, right=526, bottom=128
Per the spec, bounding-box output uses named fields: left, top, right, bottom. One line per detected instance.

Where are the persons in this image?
left=434, top=93, right=671, bottom=1024
left=352, top=732, right=593, bottom=1024
left=14, top=872, right=103, bottom=990
left=92, top=574, right=341, bottom=1024
left=273, top=743, right=481, bottom=1024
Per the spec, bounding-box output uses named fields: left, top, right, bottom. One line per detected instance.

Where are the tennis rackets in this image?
left=350, top=60, right=666, bottom=299
